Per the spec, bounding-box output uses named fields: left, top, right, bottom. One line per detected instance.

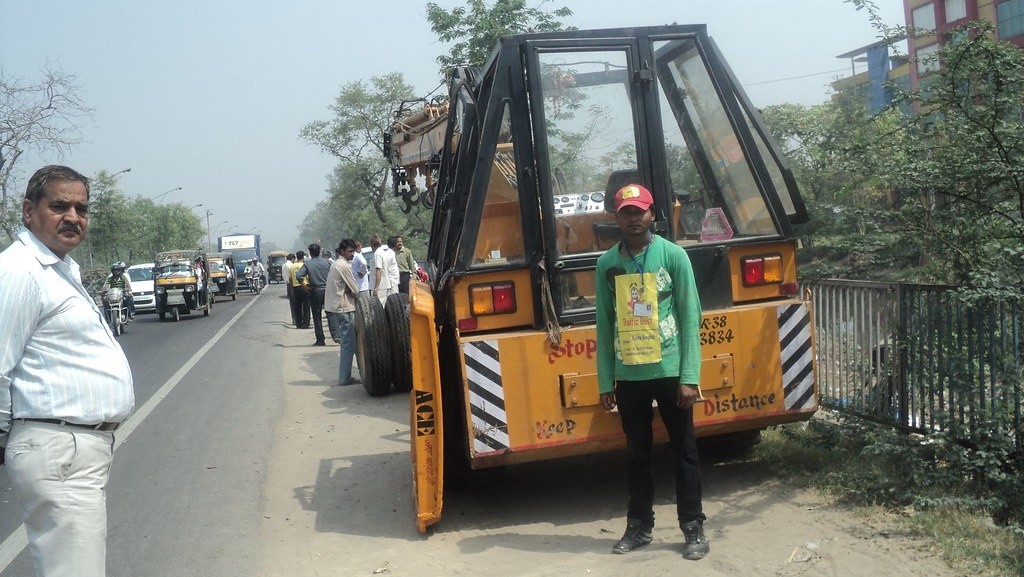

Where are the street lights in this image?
left=83, top=167, right=132, bottom=269
left=206, top=210, right=229, bottom=253
left=143, top=186, right=182, bottom=261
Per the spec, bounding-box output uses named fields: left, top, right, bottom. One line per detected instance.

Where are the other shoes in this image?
left=313, top=341, right=325, bottom=346
left=334, top=336, right=341, bottom=344
left=347, top=379, right=361, bottom=385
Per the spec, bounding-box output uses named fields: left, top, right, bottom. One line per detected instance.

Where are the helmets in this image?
left=111, top=264, right=121, bottom=270
left=252, top=257, right=257, bottom=260
left=248, top=259, right=252, bottom=262
left=116, top=261, right=126, bottom=271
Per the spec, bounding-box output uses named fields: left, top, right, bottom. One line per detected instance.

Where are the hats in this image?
left=614, top=183, right=653, bottom=213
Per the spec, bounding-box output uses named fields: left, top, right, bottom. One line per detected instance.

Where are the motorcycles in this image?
left=95, top=288, right=133, bottom=336
left=243, top=269, right=264, bottom=295
left=267, top=251, right=287, bottom=284
left=151, top=248, right=239, bottom=322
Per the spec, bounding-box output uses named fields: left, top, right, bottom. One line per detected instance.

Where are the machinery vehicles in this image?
left=350, top=20, right=821, bottom=540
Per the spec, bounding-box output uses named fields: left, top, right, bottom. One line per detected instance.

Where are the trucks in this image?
left=217, top=233, right=262, bottom=289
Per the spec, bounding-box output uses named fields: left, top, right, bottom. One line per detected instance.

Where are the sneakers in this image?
left=679, top=520, right=709, bottom=559
left=613, top=517, right=654, bottom=553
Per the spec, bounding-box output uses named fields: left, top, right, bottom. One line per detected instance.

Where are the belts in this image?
left=12, top=415, right=118, bottom=431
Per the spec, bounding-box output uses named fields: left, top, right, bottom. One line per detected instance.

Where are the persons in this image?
left=196, top=268, right=204, bottom=306
left=282, top=236, right=417, bottom=386
left=0, top=164, right=134, bottom=577
left=244, top=258, right=266, bottom=288
left=225, top=265, right=231, bottom=279
left=101, top=261, right=134, bottom=324
left=646, top=305, right=651, bottom=310
left=596, top=183, right=709, bottom=559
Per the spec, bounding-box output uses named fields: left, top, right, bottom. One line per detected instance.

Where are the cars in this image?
left=359, top=245, right=418, bottom=275
left=126, top=262, right=156, bottom=312
